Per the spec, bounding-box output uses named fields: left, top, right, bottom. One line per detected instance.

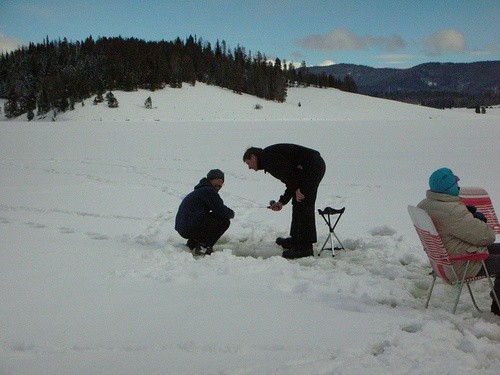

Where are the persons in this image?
left=174, top=168, right=235, bottom=257
left=243, top=143, right=326, bottom=259
left=417, top=167, right=500, bottom=316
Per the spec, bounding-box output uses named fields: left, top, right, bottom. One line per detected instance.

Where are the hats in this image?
left=207, top=169, right=225, bottom=180
left=428, top=168, right=459, bottom=195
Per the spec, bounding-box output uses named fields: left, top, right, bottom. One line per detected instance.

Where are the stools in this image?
left=317, top=207, right=347, bottom=257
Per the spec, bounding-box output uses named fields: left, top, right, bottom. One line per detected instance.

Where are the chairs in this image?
left=407, top=204, right=500, bottom=315
left=458, top=187, right=500, bottom=234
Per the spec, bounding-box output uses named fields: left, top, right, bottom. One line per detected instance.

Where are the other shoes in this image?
left=491, top=302, right=500, bottom=315
left=276, top=236, right=299, bottom=248
left=490, top=287, right=500, bottom=300
left=196, top=244, right=213, bottom=255
left=282, top=247, right=314, bottom=258
left=186, top=238, right=198, bottom=249
